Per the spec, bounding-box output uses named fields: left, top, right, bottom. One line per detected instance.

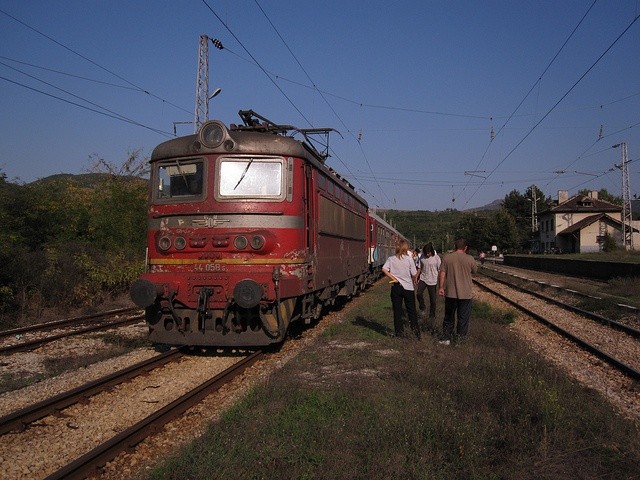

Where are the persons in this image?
left=381, top=240, right=422, bottom=338
left=479, top=251, right=486, bottom=266
left=438, top=238, right=477, bottom=346
left=412, top=248, right=422, bottom=268
left=414, top=244, right=446, bottom=319
left=409, top=251, right=420, bottom=283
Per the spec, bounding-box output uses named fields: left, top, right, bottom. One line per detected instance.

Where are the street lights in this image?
left=534, top=197, right=540, bottom=231
left=194, top=88, right=221, bottom=134
left=527, top=199, right=534, bottom=232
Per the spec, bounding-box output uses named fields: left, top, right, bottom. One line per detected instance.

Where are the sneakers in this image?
left=438, top=340, right=451, bottom=345
left=421, top=305, right=427, bottom=315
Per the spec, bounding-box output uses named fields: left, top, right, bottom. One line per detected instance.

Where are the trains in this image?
left=130, top=120, right=413, bottom=352
